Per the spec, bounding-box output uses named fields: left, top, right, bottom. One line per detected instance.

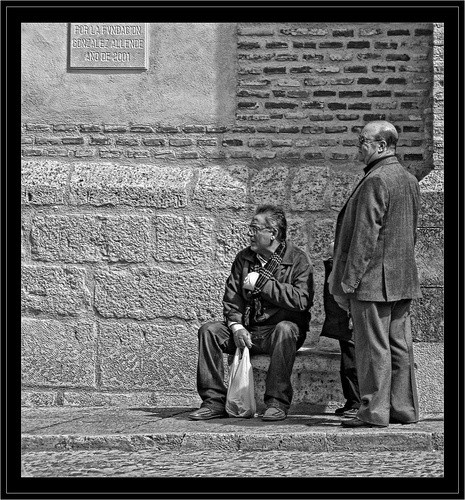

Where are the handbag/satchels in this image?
left=224, top=344, right=257, bottom=417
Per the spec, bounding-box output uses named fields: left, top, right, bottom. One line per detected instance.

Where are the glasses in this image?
left=359, top=135, right=381, bottom=144
left=248, top=225, right=269, bottom=234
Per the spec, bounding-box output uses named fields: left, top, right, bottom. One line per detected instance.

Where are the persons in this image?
left=328, top=120, right=419, bottom=427
left=188, top=203, right=314, bottom=420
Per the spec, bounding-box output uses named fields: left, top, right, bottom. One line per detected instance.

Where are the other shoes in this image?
left=262, top=407, right=287, bottom=420
left=341, top=416, right=385, bottom=427
left=188, top=405, right=227, bottom=419
left=335, top=407, right=349, bottom=413
left=343, top=408, right=358, bottom=417
